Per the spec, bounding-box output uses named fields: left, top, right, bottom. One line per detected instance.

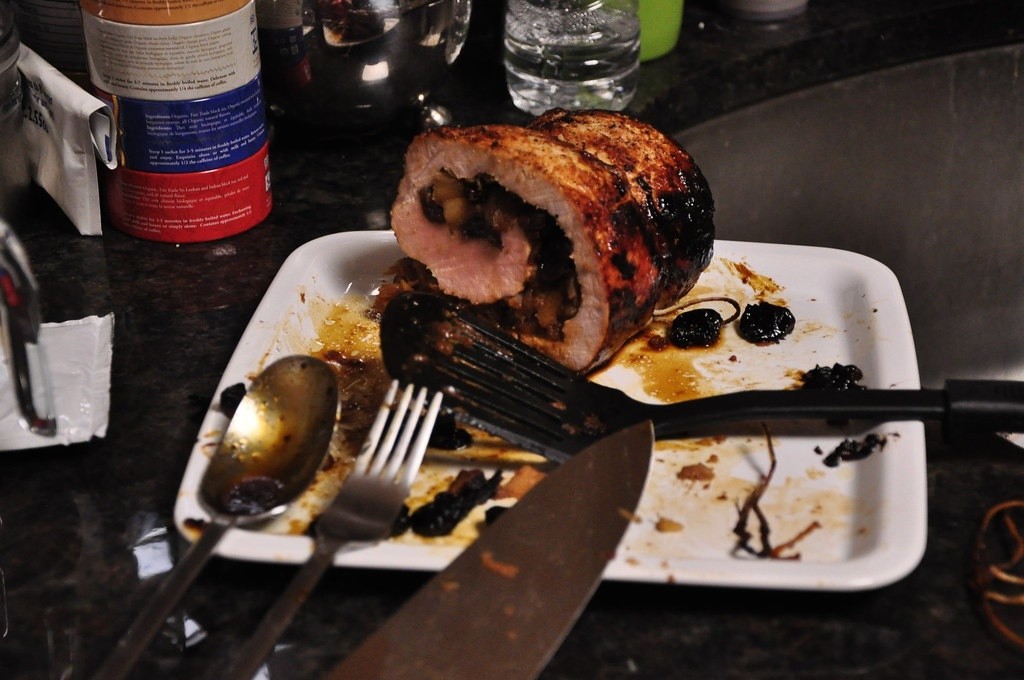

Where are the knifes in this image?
left=325, top=418, right=660, bottom=680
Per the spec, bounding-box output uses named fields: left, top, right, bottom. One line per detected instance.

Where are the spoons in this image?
left=96, top=356, right=338, bottom=680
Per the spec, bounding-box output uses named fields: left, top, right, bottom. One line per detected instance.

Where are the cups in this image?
left=0, top=0, right=29, bottom=237
left=605, top=0, right=683, bottom=64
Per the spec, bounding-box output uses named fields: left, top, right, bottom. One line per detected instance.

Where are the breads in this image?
left=376, top=106, right=716, bottom=384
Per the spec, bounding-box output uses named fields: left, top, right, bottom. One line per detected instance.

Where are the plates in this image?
left=327, top=17, right=401, bottom=46
left=178, top=229, right=931, bottom=591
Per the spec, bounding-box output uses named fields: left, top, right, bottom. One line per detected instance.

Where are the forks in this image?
left=218, top=380, right=445, bottom=680
left=452, top=307, right=1024, bottom=465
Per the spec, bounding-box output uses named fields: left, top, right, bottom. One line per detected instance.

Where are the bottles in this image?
left=505, top=0, right=638, bottom=117
left=82, top=0, right=273, bottom=245
